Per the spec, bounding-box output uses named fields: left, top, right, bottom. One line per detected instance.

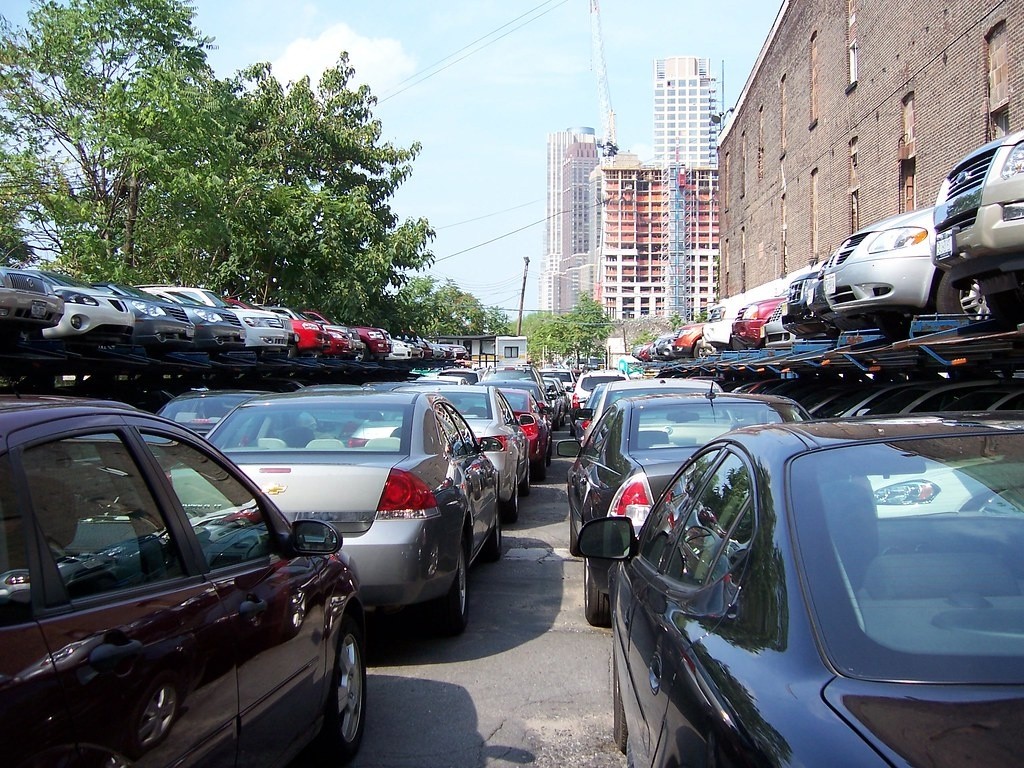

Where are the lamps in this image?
left=711, top=111, right=726, bottom=131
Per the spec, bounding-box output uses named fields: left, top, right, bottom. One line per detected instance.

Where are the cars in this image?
left=556, top=129, right=1023, bottom=632
left=0, top=263, right=585, bottom=768
left=579, top=409, right=1023, bottom=768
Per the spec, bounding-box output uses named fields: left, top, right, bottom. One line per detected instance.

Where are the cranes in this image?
left=590, top=0, right=617, bottom=167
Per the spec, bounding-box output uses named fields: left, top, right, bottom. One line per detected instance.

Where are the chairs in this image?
left=279, top=426, right=314, bottom=449
left=467, top=407, right=486, bottom=418
left=638, top=430, right=670, bottom=450
